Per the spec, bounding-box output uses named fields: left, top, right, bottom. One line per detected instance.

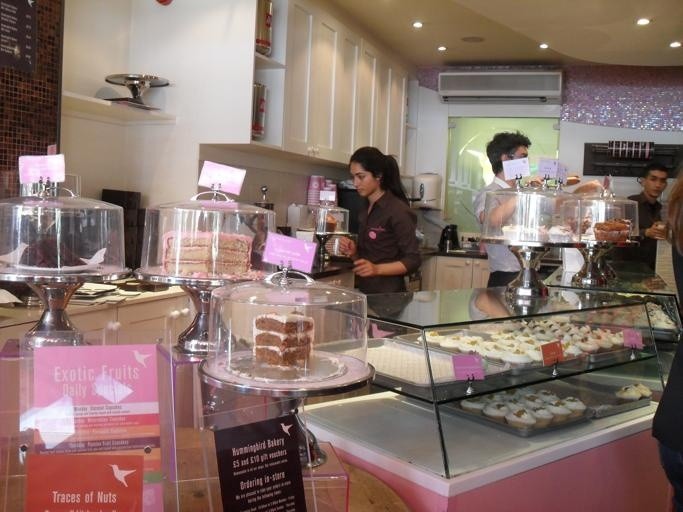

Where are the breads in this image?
left=18, top=237, right=85, bottom=268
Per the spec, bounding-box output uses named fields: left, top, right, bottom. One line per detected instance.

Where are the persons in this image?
left=652, top=172, right=683, bottom=512
left=473, top=132, right=543, bottom=288
left=338, top=147, right=421, bottom=291
left=610, top=165, right=666, bottom=278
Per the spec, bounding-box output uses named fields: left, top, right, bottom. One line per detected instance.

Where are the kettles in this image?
left=439, top=224, right=459, bottom=256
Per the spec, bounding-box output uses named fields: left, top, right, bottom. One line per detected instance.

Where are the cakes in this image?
left=160, top=232, right=252, bottom=276
left=417, top=315, right=651, bottom=429
left=501, top=216, right=630, bottom=241
left=251, top=313, right=315, bottom=368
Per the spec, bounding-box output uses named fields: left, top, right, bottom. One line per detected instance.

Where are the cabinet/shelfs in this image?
left=288, top=1, right=363, bottom=166
left=304, top=269, right=356, bottom=345
left=357, top=255, right=435, bottom=338
left=355, top=36, right=407, bottom=176
left=249, top=1, right=288, bottom=148
left=433, top=255, right=519, bottom=324
left=1, top=305, right=116, bottom=485
left=115, top=290, right=193, bottom=427
left=519, top=261, right=683, bottom=402
left=294, top=290, right=666, bottom=512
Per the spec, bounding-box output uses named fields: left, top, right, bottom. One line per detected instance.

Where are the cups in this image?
left=307, top=176, right=338, bottom=207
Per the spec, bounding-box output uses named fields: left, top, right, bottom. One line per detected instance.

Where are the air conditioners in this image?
left=438, top=69, right=562, bottom=104
